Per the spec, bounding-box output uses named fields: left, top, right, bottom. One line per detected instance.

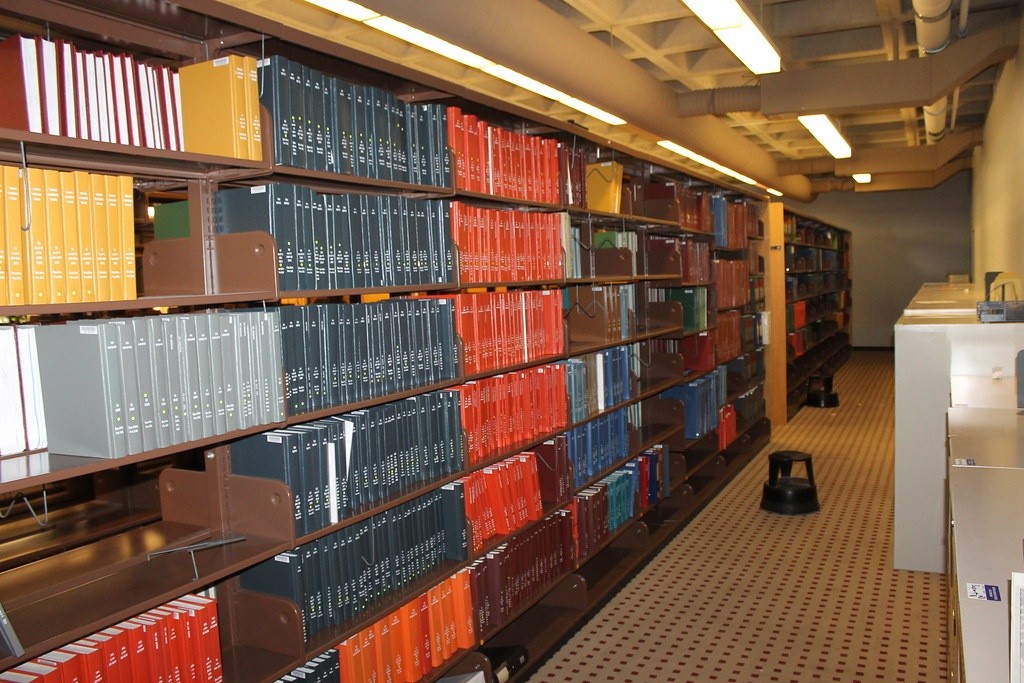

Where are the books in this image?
left=0, top=34, right=770, bottom=683
left=786, top=216, right=851, bottom=366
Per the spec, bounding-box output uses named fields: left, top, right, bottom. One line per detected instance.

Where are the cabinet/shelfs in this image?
left=0, top=0, right=851, bottom=683
left=894, top=281, right=1024, bottom=683
